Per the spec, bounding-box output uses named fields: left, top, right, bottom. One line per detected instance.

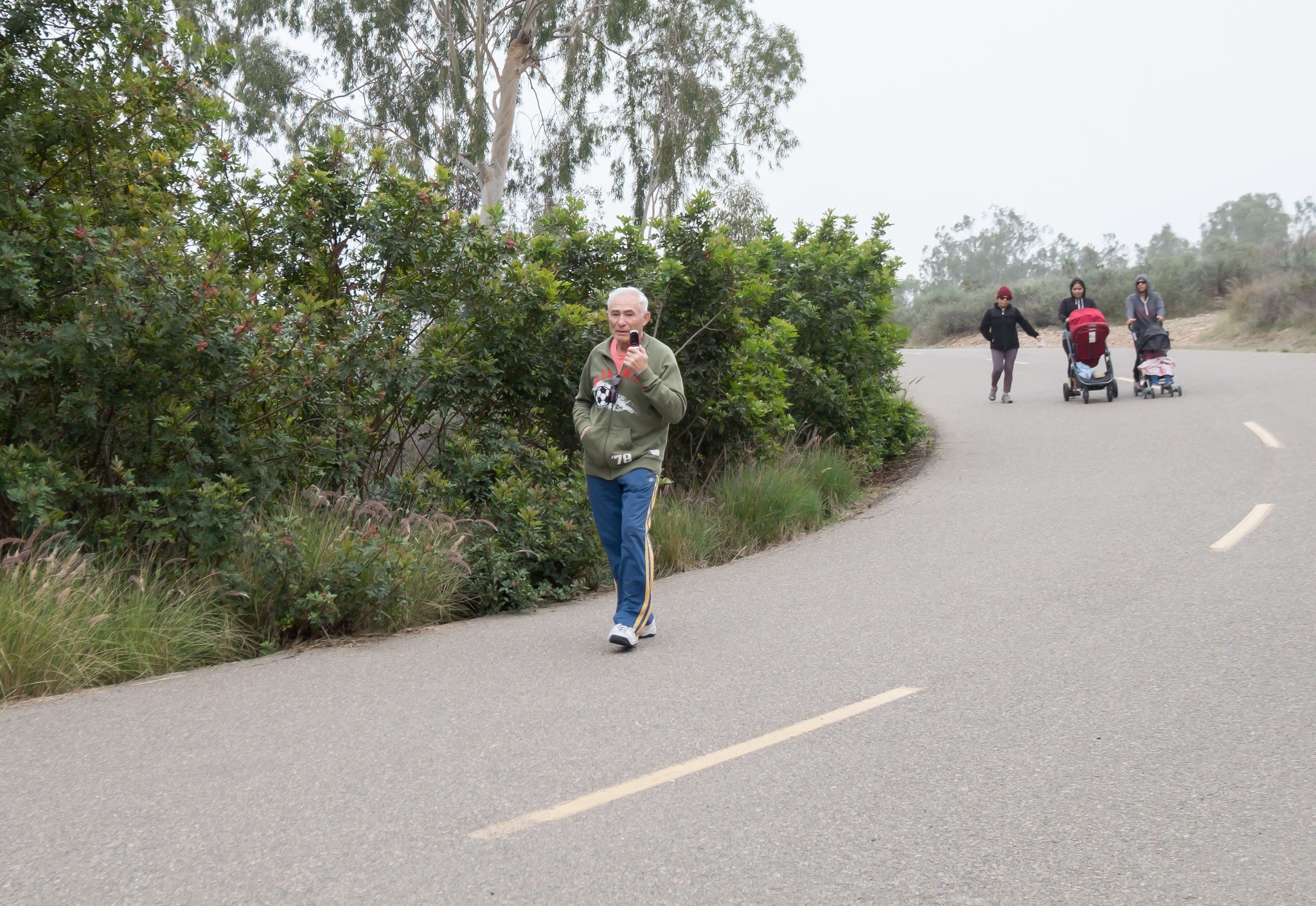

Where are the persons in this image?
left=1125, top=273, right=1165, bottom=393
left=979, top=286, right=1041, bottom=403
left=1058, top=278, right=1097, bottom=399
left=571, top=287, right=687, bottom=648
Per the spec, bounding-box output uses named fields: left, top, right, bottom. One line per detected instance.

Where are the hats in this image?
left=996, top=286, right=1011, bottom=299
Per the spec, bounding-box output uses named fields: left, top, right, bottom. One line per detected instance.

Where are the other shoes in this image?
left=1133, top=382, right=1140, bottom=388
left=1074, top=391, right=1081, bottom=396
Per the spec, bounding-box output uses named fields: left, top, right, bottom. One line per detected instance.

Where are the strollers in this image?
left=1127, top=319, right=1182, bottom=400
left=1062, top=308, right=1120, bottom=404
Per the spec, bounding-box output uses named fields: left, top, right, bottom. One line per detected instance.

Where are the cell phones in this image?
left=630, top=330, right=639, bottom=347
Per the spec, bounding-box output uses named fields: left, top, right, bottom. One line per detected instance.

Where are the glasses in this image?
left=999, top=295, right=1012, bottom=301
left=1136, top=281, right=1146, bottom=285
left=608, top=375, right=621, bottom=404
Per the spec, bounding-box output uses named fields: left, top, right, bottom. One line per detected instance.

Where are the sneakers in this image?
left=988, top=385, right=998, bottom=401
left=606, top=623, right=639, bottom=646
left=1001, top=394, right=1013, bottom=403
left=639, top=619, right=657, bottom=638
